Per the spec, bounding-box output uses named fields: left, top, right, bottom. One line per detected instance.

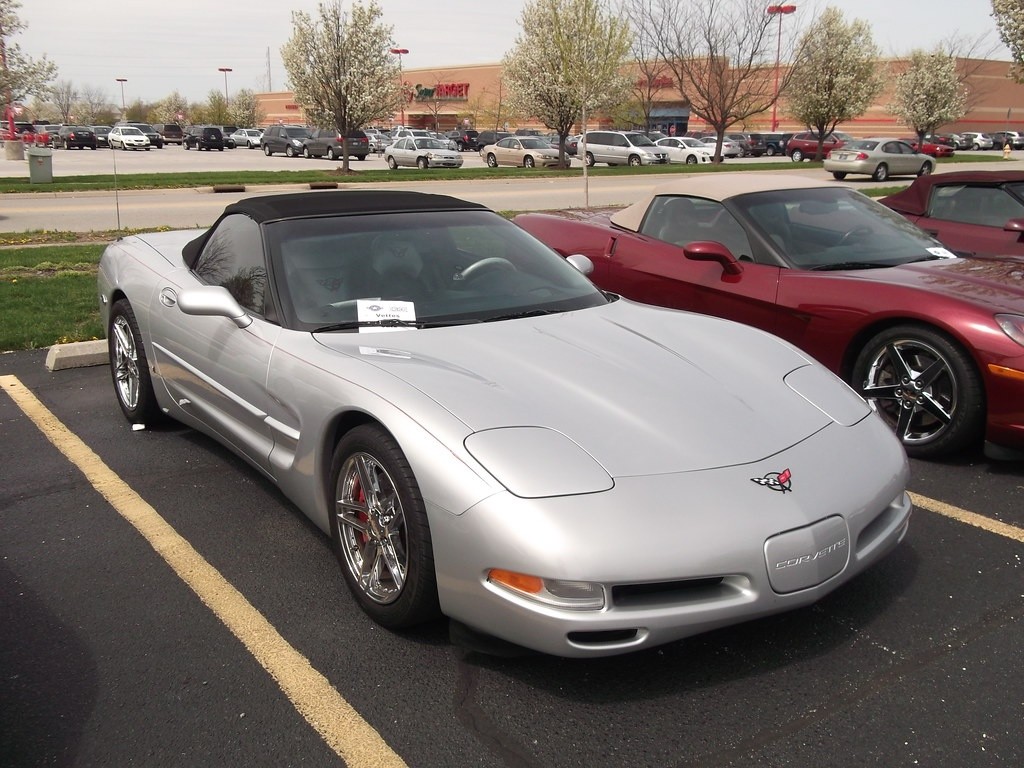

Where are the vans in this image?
left=574, top=131, right=670, bottom=167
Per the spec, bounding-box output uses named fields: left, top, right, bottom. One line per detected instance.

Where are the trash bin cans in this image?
left=28, top=148, right=53, bottom=184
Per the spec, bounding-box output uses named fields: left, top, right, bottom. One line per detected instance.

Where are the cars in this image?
left=884, top=131, right=1024, bottom=158
left=97, top=189, right=913, bottom=658
left=788, top=170, right=1024, bottom=256
left=823, top=138, right=937, bottom=182
left=632, top=130, right=794, bottom=165
left=509, top=173, right=1024, bottom=460
left=482, top=136, right=572, bottom=168
left=0, top=120, right=238, bottom=150
left=364, top=125, right=582, bottom=155
left=385, top=137, right=464, bottom=169
left=230, top=129, right=264, bottom=149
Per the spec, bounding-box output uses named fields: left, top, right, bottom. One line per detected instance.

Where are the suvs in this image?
left=786, top=129, right=871, bottom=163
left=256, top=121, right=369, bottom=161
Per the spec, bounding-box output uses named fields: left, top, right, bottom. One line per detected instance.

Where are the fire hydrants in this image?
left=1003, top=144, right=1012, bottom=160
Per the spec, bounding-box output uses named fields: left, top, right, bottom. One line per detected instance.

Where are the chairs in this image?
left=419, top=141, right=428, bottom=147
left=373, top=234, right=434, bottom=305
left=657, top=197, right=700, bottom=247
left=752, top=202, right=794, bottom=257
left=285, top=251, right=357, bottom=319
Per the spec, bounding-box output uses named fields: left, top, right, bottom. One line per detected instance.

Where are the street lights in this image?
left=768, top=5, right=796, bottom=132
left=219, top=69, right=233, bottom=108
left=117, top=79, right=127, bottom=108
left=391, top=49, right=409, bottom=129
